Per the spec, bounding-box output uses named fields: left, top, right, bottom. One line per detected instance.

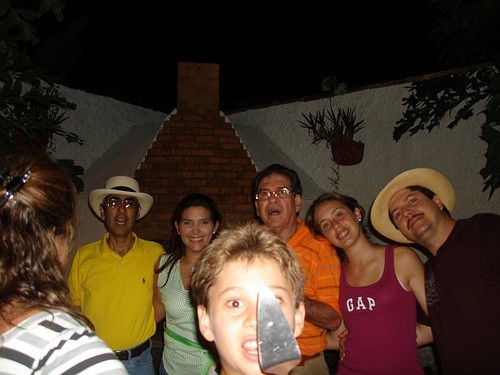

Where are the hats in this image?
left=89, top=175, right=154, bottom=220
left=370, top=166, right=456, bottom=243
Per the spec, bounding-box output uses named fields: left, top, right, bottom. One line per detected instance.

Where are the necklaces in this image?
left=185, top=256, right=197, bottom=276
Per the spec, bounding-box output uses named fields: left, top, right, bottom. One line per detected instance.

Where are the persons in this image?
left=191, top=222, right=305, bottom=375
left=339, top=167, right=500, bottom=375
left=0, top=145, right=128, bottom=375
left=64, top=176, right=168, bottom=375
left=152, top=193, right=220, bottom=375
left=305, top=193, right=428, bottom=375
left=254, top=164, right=341, bottom=375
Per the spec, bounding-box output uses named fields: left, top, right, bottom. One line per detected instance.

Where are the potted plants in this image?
left=297, top=105, right=366, bottom=167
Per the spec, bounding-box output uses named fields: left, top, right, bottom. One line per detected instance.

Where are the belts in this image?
left=113, top=338, right=151, bottom=360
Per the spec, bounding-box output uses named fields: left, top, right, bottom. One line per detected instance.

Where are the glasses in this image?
left=107, top=196, right=139, bottom=209
left=254, top=186, right=295, bottom=201
